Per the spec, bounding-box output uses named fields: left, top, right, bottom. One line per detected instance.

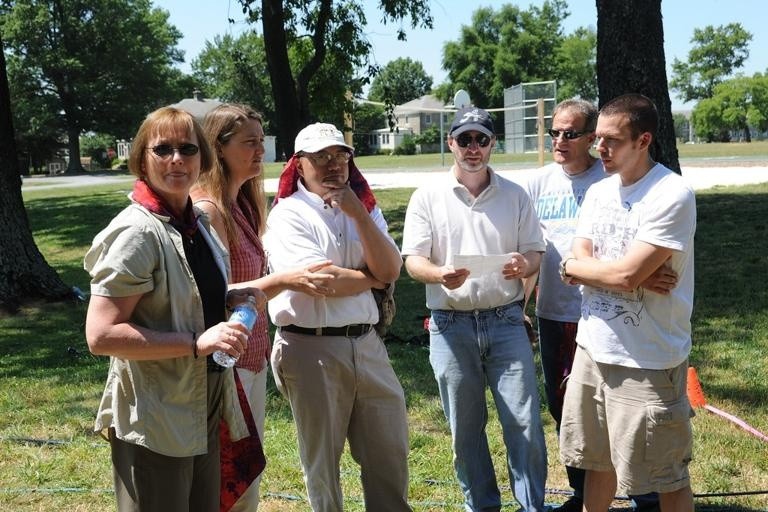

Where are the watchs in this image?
left=558, top=257, right=576, bottom=282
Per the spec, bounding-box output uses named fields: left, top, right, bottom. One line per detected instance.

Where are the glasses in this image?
left=307, top=150, right=351, bottom=163
left=547, top=127, right=594, bottom=140
left=453, top=132, right=491, bottom=148
left=140, top=143, right=202, bottom=157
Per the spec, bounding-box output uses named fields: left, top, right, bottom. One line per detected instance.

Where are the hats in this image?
left=449, top=106, right=495, bottom=140
left=294, top=121, right=355, bottom=153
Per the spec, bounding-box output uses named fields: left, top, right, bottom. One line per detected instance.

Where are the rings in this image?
left=332, top=200, right=338, bottom=205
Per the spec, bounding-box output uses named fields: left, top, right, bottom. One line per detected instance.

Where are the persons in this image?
left=81, top=105, right=252, bottom=511
left=188, top=107, right=337, bottom=510
left=260, top=121, right=410, bottom=512
left=508, top=97, right=663, bottom=509
left=557, top=92, right=700, bottom=511
left=400, top=106, right=551, bottom=510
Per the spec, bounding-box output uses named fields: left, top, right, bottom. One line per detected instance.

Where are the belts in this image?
left=277, top=323, right=377, bottom=338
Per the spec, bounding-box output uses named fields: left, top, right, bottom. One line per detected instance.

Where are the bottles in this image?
left=210, top=294, right=258, bottom=368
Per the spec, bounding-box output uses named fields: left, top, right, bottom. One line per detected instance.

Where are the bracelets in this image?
left=188, top=330, right=201, bottom=361
